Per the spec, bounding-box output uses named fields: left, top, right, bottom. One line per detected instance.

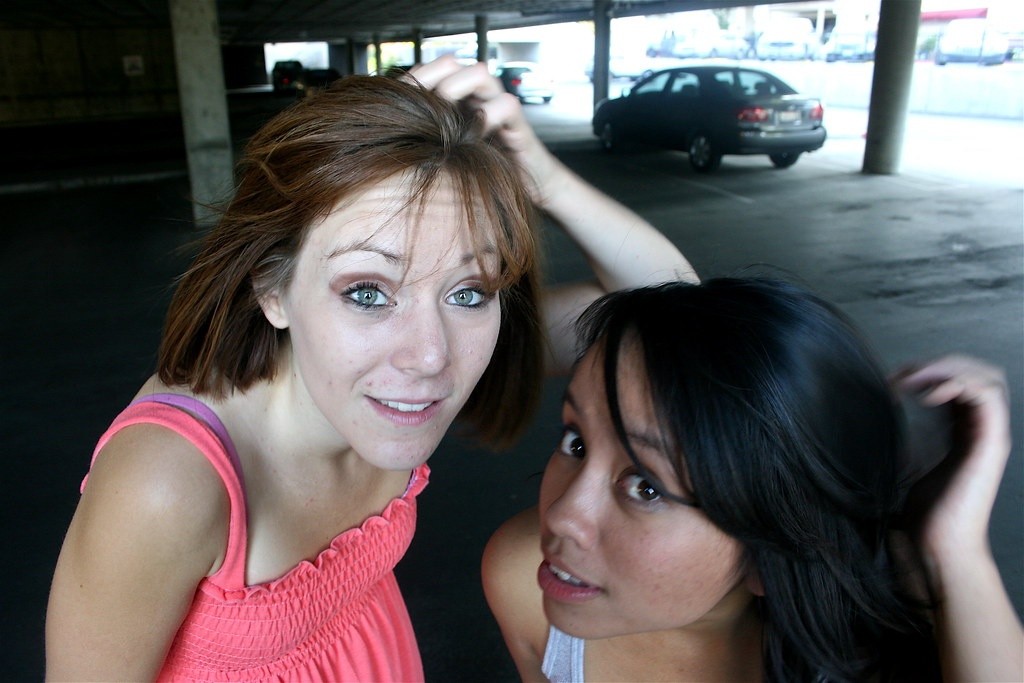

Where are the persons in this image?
left=42, top=54, right=700, bottom=683
left=479, top=276, right=1024, bottom=683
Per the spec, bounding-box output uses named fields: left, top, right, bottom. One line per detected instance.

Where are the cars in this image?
left=754, top=17, right=818, bottom=61
left=273, top=62, right=343, bottom=101
left=489, top=62, right=554, bottom=105
left=919, top=17, right=1024, bottom=69
left=591, top=62, right=827, bottom=174
left=645, top=29, right=749, bottom=61
left=824, top=22, right=879, bottom=61
